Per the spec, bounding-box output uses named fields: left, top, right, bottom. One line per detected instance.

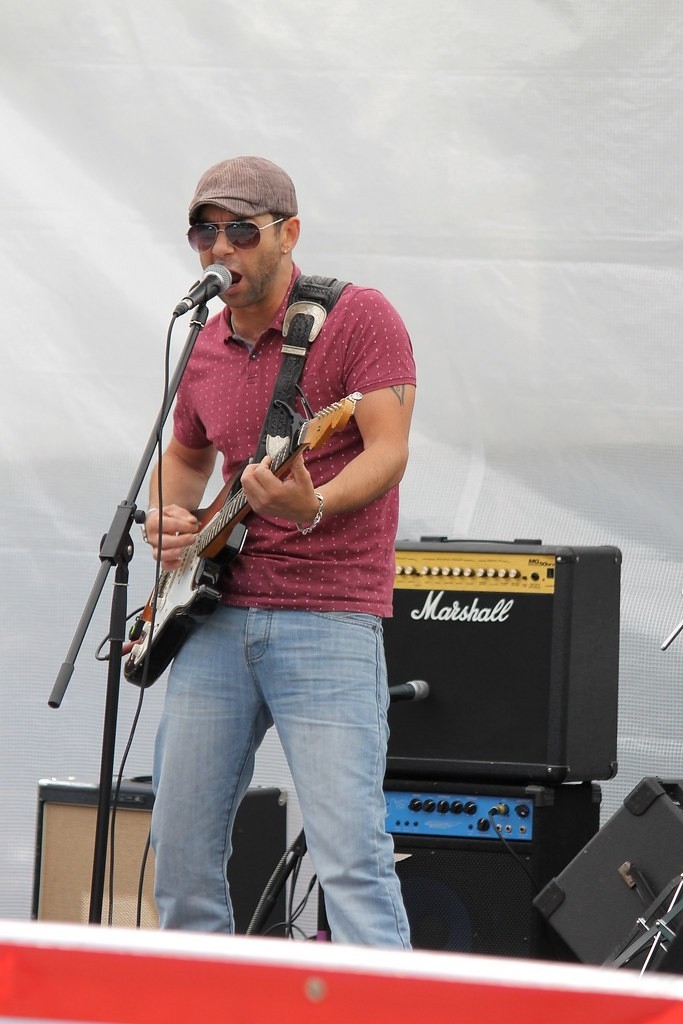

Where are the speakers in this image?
left=316, top=535, right=683, bottom=976
left=30, top=774, right=288, bottom=938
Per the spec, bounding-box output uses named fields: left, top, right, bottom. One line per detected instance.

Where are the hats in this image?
left=189, top=156, right=298, bottom=225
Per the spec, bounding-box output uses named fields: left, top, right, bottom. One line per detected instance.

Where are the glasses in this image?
left=185, top=218, right=288, bottom=254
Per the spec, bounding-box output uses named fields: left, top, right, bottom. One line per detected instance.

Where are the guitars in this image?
left=121, top=392, right=368, bottom=689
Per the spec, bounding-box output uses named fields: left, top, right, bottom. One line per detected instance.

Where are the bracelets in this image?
left=295, top=493, right=324, bottom=536
left=141, top=509, right=158, bottom=542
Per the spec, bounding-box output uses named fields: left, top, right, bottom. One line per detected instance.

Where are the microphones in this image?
left=173, top=264, right=232, bottom=318
left=389, top=680, right=429, bottom=703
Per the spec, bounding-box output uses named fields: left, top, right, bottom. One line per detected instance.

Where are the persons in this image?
left=152, top=156, right=416, bottom=952
